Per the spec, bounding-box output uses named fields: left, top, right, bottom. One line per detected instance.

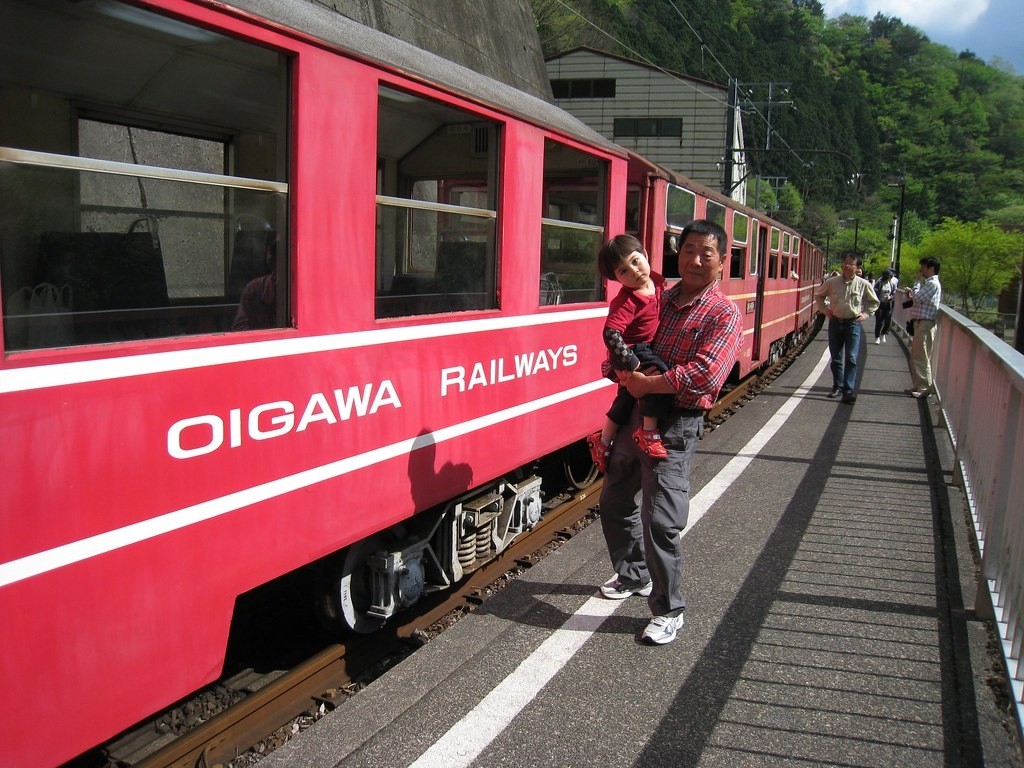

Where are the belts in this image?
left=832, top=314, right=861, bottom=324
left=913, top=318, right=933, bottom=322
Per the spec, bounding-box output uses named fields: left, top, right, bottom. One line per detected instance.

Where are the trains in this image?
left=1, top=0, right=827, bottom=766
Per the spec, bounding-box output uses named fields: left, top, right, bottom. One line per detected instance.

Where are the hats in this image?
left=881, top=271, right=896, bottom=280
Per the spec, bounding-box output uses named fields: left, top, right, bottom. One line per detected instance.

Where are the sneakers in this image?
left=874, top=337, right=881, bottom=345
left=598, top=578, right=654, bottom=599
left=634, top=425, right=668, bottom=457
left=912, top=389, right=932, bottom=399
left=879, top=333, right=887, bottom=343
left=903, top=387, right=919, bottom=393
left=586, top=431, right=608, bottom=474
left=642, top=612, right=685, bottom=644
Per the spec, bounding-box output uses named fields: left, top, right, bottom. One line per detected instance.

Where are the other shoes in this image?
left=828, top=389, right=843, bottom=398
left=842, top=394, right=855, bottom=403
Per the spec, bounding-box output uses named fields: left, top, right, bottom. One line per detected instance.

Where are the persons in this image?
left=822, top=264, right=876, bottom=291
left=230, top=234, right=279, bottom=330
left=814, top=250, right=881, bottom=403
left=884, top=267, right=899, bottom=335
left=586, top=234, right=668, bottom=473
left=596, top=220, right=745, bottom=646
left=905, top=254, right=942, bottom=399
left=874, top=270, right=896, bottom=346
left=789, top=270, right=800, bottom=281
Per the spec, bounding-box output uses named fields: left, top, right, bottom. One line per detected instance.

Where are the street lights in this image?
left=886, top=175, right=908, bottom=290
left=849, top=217, right=859, bottom=256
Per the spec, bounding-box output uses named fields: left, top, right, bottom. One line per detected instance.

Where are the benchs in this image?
left=28, top=226, right=489, bottom=338
